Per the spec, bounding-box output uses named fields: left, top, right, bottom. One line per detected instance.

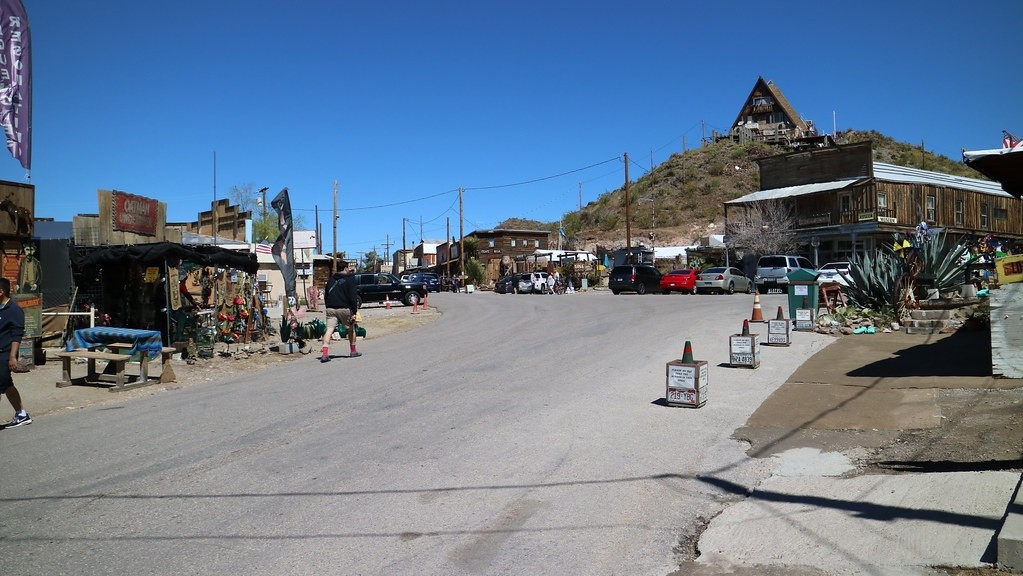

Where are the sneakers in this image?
left=5, top=413, right=32, bottom=428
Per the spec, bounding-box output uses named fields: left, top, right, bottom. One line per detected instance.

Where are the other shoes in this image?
left=350, top=351, right=363, bottom=358
left=320, top=355, right=331, bottom=363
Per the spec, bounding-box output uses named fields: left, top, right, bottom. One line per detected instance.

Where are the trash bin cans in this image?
left=786, top=268, right=821, bottom=320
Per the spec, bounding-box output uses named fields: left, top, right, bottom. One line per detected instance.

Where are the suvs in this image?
left=753, top=255, right=818, bottom=294
left=352, top=272, right=441, bottom=308
left=608, top=265, right=667, bottom=295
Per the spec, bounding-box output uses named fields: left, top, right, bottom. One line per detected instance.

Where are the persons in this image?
left=176, top=275, right=198, bottom=309
left=0, top=277, right=33, bottom=429
left=452, top=274, right=460, bottom=293
left=546, top=271, right=558, bottom=295
left=530, top=271, right=537, bottom=295
left=320, top=260, right=362, bottom=363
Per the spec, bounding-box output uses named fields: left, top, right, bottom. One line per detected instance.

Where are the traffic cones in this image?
left=410, top=298, right=420, bottom=314
left=749, top=291, right=766, bottom=322
left=768, top=306, right=792, bottom=347
left=422, top=295, right=428, bottom=310
left=665, top=341, right=709, bottom=408
left=385, top=293, right=392, bottom=309
left=729, top=318, right=761, bottom=370
left=795, top=297, right=816, bottom=332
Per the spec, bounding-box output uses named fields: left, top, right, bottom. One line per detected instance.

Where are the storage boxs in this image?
left=996, top=255, right=1023, bottom=285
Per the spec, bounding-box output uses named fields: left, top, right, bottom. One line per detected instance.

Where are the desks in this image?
left=66, top=326, right=163, bottom=387
left=819, top=282, right=848, bottom=315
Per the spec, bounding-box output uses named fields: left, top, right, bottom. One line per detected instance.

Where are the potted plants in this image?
left=277, top=314, right=299, bottom=354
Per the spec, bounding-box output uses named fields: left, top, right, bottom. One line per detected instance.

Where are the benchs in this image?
left=54, top=351, right=142, bottom=393
left=105, top=342, right=178, bottom=385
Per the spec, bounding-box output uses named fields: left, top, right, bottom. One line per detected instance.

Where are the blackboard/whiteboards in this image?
left=12, top=293, right=43, bottom=336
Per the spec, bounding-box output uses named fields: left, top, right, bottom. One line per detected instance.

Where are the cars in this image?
left=495, top=272, right=550, bottom=294
left=660, top=269, right=702, bottom=295
left=818, top=261, right=856, bottom=287
left=696, top=266, right=753, bottom=295
